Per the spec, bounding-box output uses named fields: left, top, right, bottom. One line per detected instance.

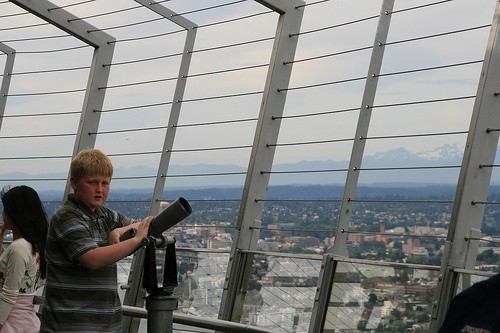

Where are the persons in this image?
left=0, top=186, right=47, bottom=332
left=39, top=149, right=155, bottom=333
left=439, top=273, right=499, bottom=333
